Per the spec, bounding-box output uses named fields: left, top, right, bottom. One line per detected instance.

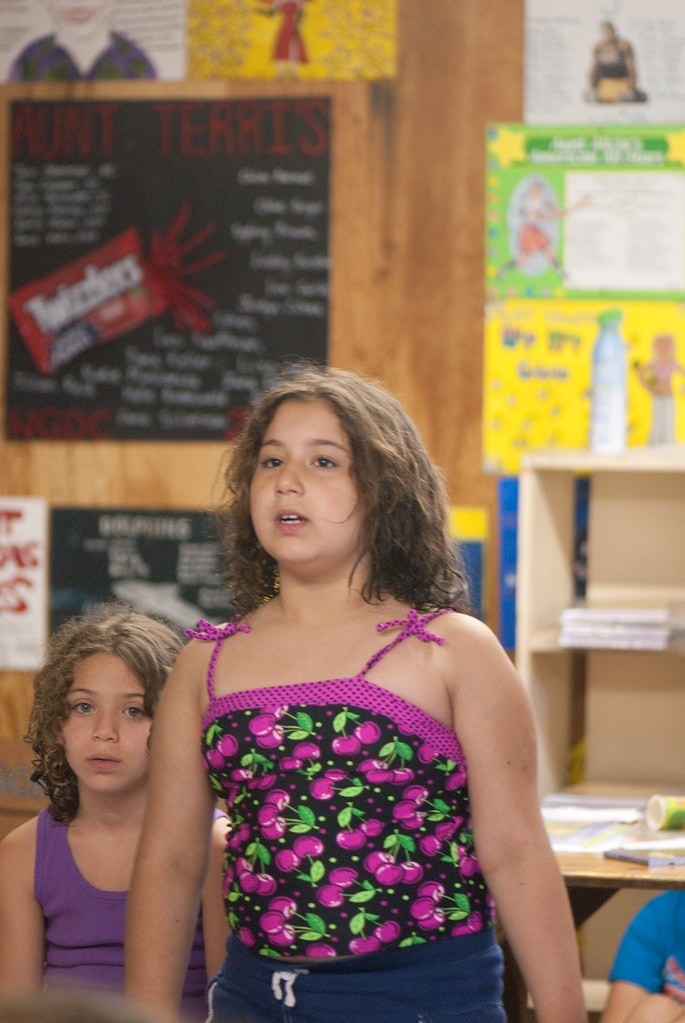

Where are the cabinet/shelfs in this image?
left=512, top=450, right=685, bottom=890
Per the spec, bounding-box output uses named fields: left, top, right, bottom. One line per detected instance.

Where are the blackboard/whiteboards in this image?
left=1, top=82, right=378, bottom=512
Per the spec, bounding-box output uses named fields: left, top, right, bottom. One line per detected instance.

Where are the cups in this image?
left=645, top=794, right=685, bottom=830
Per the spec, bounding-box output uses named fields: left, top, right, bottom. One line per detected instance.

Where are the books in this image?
left=604, top=847, right=685, bottom=866
left=557, top=597, right=676, bottom=650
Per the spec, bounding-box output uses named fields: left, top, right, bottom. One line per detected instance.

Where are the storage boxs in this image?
left=484, top=297, right=685, bottom=480
left=479, top=116, right=685, bottom=301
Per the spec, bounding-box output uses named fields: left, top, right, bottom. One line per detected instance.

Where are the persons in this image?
left=586, top=22, right=636, bottom=103
left=1, top=610, right=232, bottom=1023
left=123, top=366, right=591, bottom=1023
left=601, top=888, right=685, bottom=1023
